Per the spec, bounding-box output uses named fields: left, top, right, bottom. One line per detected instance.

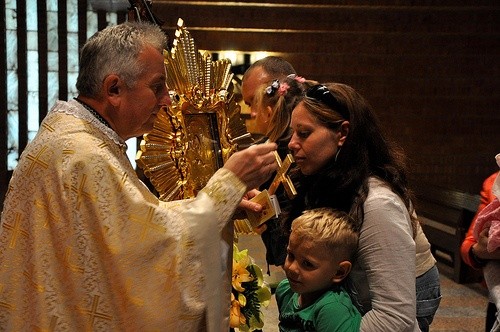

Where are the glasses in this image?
left=303, top=84, right=351, bottom=120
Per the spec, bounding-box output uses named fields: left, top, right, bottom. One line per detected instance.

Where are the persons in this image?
left=0, top=21, right=279, bottom=332
left=243, top=54, right=500, bottom=332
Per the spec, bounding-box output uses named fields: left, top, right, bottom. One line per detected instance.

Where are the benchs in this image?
left=426, top=201, right=482, bottom=284
